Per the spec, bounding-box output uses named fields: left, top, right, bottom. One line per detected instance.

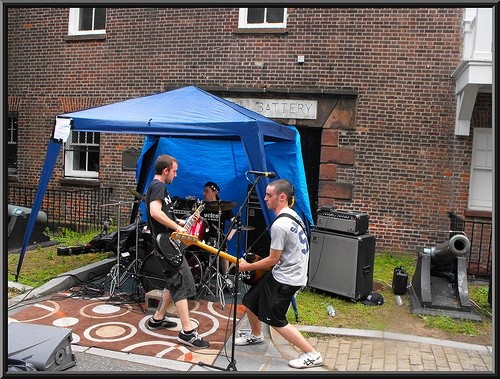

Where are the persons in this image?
left=146, top=154, right=209, bottom=349
left=235, top=179, right=323, bottom=369
left=203, top=182, right=237, bottom=288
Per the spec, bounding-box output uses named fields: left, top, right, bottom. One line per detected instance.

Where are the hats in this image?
left=204, top=182, right=221, bottom=200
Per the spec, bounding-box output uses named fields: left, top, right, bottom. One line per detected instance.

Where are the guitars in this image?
left=154, top=202, right=207, bottom=267
left=170, top=231, right=268, bottom=287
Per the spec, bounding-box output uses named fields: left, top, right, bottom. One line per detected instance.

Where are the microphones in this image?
left=247, top=170, right=276, bottom=178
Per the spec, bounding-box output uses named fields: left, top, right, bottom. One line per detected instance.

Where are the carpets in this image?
left=9, top=290, right=247, bottom=366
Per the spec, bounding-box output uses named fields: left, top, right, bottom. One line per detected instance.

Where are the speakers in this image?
left=6, top=320, right=76, bottom=372
left=308, top=227, right=377, bottom=301
left=244, top=205, right=272, bottom=286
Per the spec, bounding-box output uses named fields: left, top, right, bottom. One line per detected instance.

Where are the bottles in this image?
left=396, top=295, right=403, bottom=306
left=326, top=304, right=335, bottom=317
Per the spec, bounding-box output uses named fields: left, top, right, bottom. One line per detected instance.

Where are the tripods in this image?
left=99, top=198, right=143, bottom=303
left=194, top=204, right=236, bottom=310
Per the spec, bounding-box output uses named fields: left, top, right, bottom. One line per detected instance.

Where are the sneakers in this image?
left=235, top=331, right=264, bottom=346
left=178, top=329, right=210, bottom=349
left=288, top=352, right=323, bottom=369
left=148, top=317, right=178, bottom=330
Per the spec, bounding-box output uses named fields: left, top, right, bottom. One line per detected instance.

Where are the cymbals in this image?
left=200, top=199, right=238, bottom=211
left=226, top=226, right=256, bottom=231
left=100, top=200, right=140, bottom=206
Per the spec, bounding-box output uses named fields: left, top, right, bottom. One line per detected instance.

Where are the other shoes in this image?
left=224, top=278, right=233, bottom=288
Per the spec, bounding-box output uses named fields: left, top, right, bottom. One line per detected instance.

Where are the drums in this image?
left=137, top=247, right=202, bottom=306
left=175, top=213, right=211, bottom=243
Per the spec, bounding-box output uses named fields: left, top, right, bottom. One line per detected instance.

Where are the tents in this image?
left=15, top=85, right=313, bottom=282
